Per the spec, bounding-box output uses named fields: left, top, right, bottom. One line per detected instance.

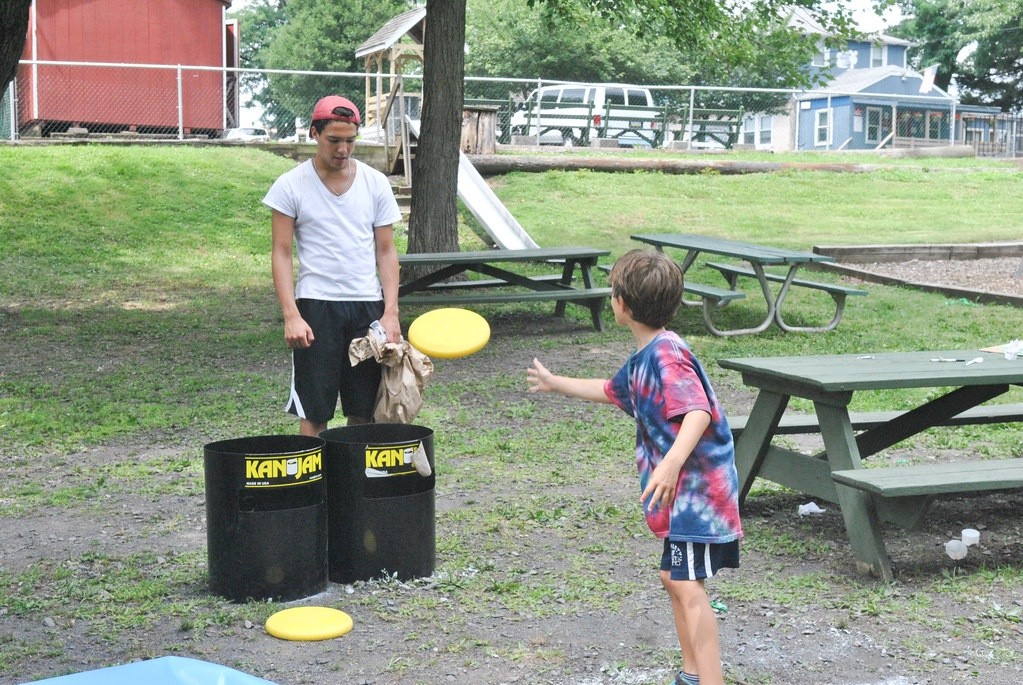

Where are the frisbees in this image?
left=408, top=308, right=491, bottom=359
left=265, top=607, right=353, bottom=640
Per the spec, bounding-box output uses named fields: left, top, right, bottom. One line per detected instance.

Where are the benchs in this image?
left=724, top=402, right=1023, bottom=435
left=597, top=265, right=746, bottom=336
left=594, top=99, right=672, bottom=149
left=397, top=286, right=615, bottom=332
left=706, top=261, right=868, bottom=330
left=672, top=104, right=744, bottom=150
left=832, top=456, right=1022, bottom=532
left=427, top=274, right=576, bottom=291
left=465, top=97, right=516, bottom=145
left=520, top=97, right=596, bottom=147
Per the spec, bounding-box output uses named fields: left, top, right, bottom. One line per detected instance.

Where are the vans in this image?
left=507, top=82, right=662, bottom=147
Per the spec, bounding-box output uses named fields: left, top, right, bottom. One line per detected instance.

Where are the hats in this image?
left=309, top=96, right=363, bottom=138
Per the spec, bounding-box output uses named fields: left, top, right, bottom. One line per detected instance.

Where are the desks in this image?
left=630, top=232, right=834, bottom=332
left=395, top=244, right=611, bottom=332
left=717, top=346, right=1023, bottom=581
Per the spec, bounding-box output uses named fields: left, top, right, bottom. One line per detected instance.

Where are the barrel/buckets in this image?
left=204, top=433, right=330, bottom=603
left=318, top=421, right=440, bottom=591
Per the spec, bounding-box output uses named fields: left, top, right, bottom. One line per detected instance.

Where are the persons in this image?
left=261, top=93, right=403, bottom=441
left=524, top=250, right=743, bottom=685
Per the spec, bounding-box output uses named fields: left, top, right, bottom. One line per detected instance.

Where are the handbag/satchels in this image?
left=348, top=328, right=435, bottom=425
